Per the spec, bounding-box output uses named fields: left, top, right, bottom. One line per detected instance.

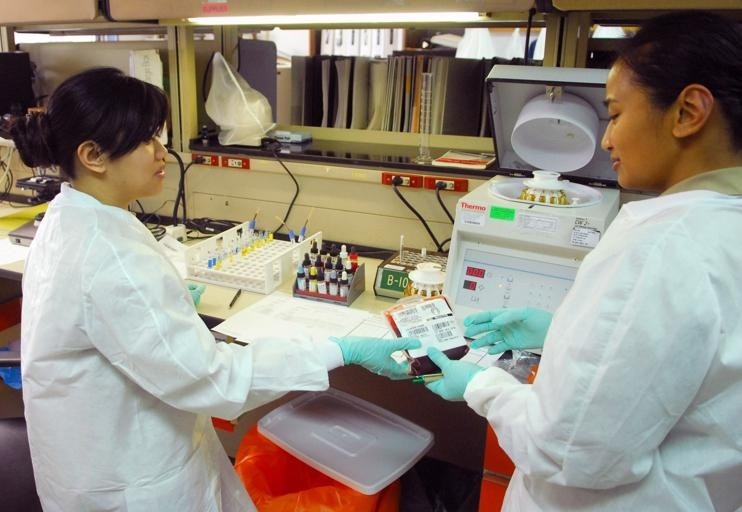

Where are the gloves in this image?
left=421, top=350, right=486, bottom=405
left=186, top=281, right=208, bottom=310
left=461, top=304, right=558, bottom=357
left=328, top=330, right=422, bottom=388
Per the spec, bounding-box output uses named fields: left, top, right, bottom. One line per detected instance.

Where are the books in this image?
left=288, top=46, right=497, bottom=171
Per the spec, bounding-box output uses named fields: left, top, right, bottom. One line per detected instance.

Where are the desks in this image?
left=0, top=197, right=540, bottom=384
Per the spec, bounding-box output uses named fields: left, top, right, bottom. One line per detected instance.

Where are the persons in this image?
left=425, top=6, right=742, bottom=512
left=9, top=64, right=424, bottom=512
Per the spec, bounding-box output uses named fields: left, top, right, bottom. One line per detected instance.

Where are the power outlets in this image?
left=192, top=153, right=250, bottom=169
left=381, top=172, right=469, bottom=193
